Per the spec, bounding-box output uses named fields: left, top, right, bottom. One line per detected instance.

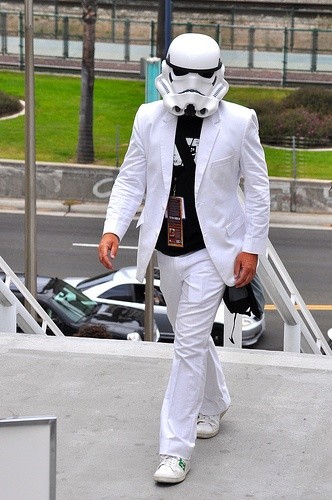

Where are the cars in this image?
left=62, top=267, right=266, bottom=346
left=1, top=272, right=159, bottom=343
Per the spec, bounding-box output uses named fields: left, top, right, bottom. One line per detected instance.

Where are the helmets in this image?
left=155, top=32, right=229, bottom=117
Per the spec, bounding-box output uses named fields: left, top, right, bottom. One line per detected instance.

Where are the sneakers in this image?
left=152, top=454, right=191, bottom=484
left=196, top=405, right=228, bottom=439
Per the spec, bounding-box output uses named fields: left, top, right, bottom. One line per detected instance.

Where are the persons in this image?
left=98, top=33, right=271, bottom=483
